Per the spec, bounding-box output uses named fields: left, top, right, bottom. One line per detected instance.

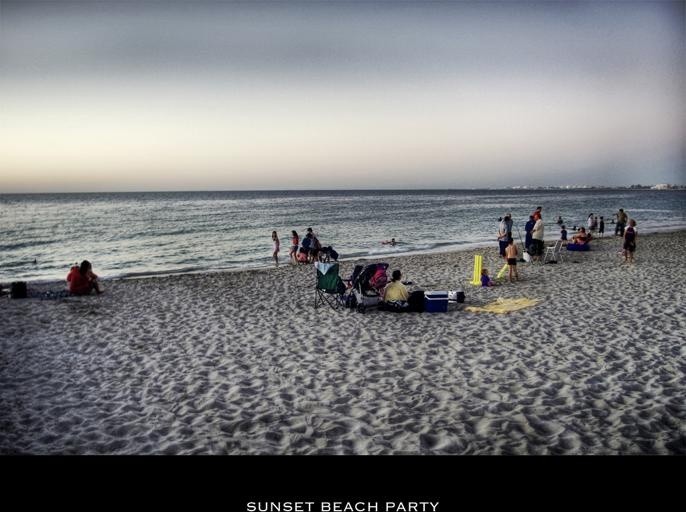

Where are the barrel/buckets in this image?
left=523, top=251, right=531, bottom=263
left=448, top=290, right=457, bottom=302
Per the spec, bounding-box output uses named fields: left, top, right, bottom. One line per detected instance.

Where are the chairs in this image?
left=313, top=261, right=347, bottom=310
left=543, top=240, right=564, bottom=263
left=340, top=265, right=364, bottom=288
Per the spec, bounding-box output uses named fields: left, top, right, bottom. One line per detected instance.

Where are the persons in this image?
left=67, top=260, right=104, bottom=296
left=560, top=225, right=567, bottom=241
left=615, top=209, right=628, bottom=237
left=271, top=231, right=280, bottom=267
left=505, top=238, right=519, bottom=282
left=557, top=216, right=562, bottom=224
left=572, top=213, right=604, bottom=245
left=288, top=228, right=320, bottom=267
left=623, top=219, right=636, bottom=262
left=383, top=269, right=410, bottom=308
left=481, top=269, right=493, bottom=286
left=498, top=206, right=545, bottom=263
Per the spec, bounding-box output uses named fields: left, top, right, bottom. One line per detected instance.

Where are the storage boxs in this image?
left=424, top=289, right=449, bottom=312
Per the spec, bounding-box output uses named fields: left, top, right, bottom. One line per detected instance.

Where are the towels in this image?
left=30, top=288, right=71, bottom=300
left=465, top=297, right=546, bottom=313
